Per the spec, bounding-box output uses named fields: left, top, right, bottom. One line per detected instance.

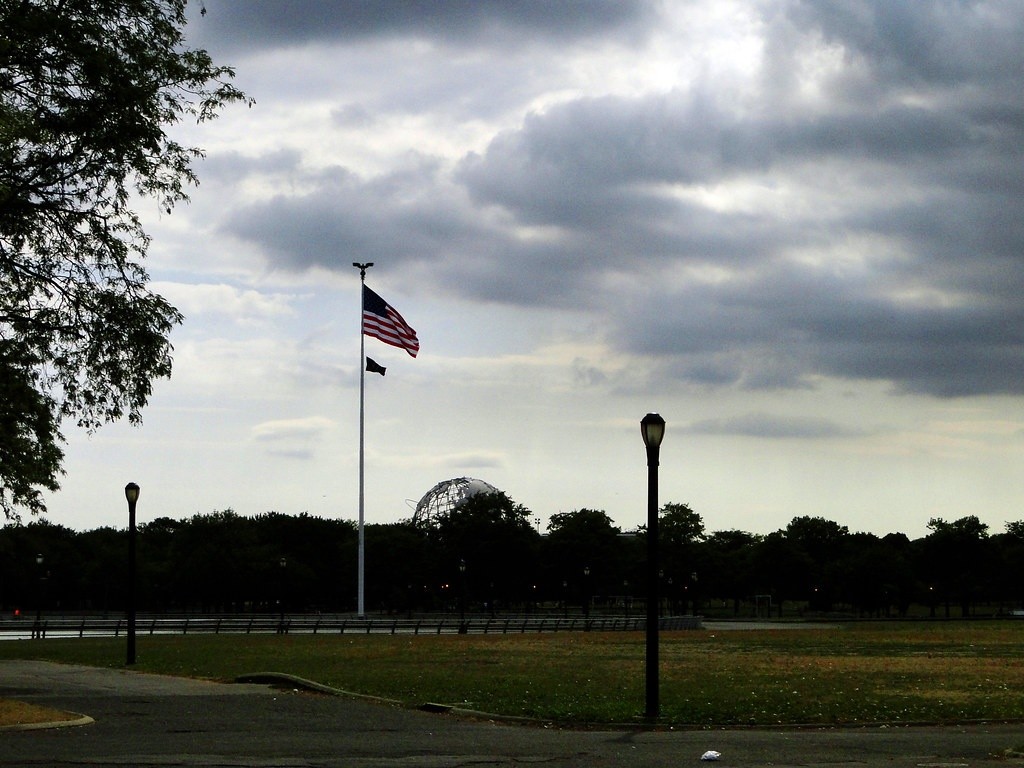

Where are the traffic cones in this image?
left=659, top=569, right=664, bottom=618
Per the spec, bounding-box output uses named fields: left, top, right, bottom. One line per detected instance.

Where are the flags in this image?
left=365, top=356, right=387, bottom=377
left=361, top=282, right=419, bottom=358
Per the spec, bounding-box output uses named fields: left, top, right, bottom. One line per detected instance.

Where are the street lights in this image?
left=639, top=411, right=666, bottom=726
left=584, top=567, right=591, bottom=627
left=35, top=553, right=44, bottom=639
left=459, top=563, right=467, bottom=626
left=692, top=573, right=699, bottom=616
left=280, top=557, right=287, bottom=625
left=125, top=482, right=141, bottom=664
